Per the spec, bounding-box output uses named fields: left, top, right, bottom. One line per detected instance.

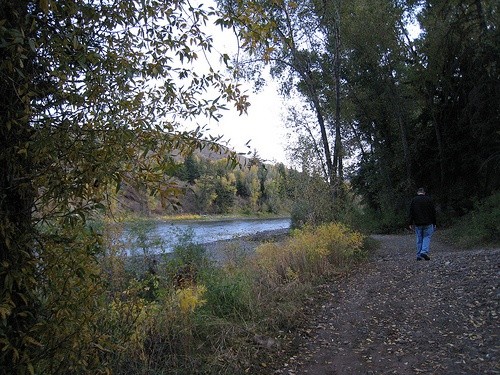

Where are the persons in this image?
left=406, top=187, right=436, bottom=261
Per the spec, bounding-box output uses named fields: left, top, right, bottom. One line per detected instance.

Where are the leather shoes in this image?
left=417, top=256, right=422, bottom=260
left=420, top=253, right=430, bottom=261
